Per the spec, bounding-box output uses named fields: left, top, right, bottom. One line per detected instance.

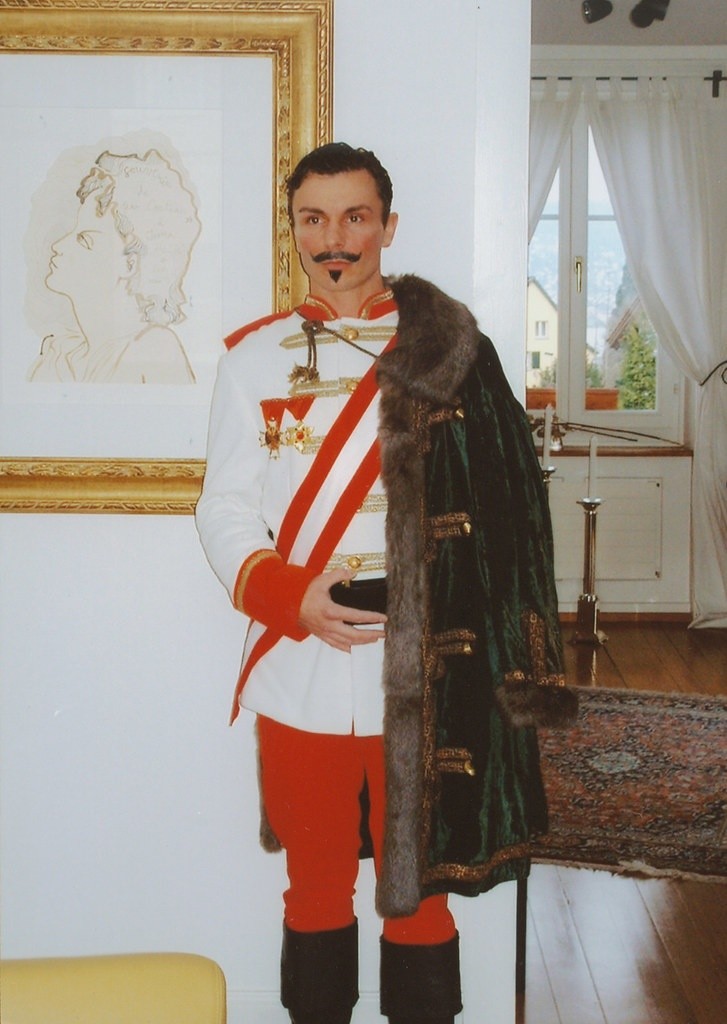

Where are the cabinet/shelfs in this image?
left=539, top=457, right=694, bottom=621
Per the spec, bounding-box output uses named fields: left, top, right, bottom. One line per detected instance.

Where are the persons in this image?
left=195, top=142, right=565, bottom=1024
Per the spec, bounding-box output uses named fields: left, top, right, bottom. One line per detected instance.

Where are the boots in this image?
left=380, top=929, right=463, bottom=1024
left=280, top=917, right=359, bottom=1024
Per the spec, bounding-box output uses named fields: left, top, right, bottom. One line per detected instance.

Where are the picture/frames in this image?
left=2, top=1, right=335, bottom=514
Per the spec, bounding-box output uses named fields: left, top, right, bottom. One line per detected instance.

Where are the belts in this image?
left=331, top=578, right=388, bottom=625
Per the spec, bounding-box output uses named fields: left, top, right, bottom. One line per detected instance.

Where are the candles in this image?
left=543, top=403, right=553, bottom=468
left=589, top=436, right=598, bottom=501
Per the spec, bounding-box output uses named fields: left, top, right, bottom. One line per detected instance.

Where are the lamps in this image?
left=582, top=0, right=613, bottom=24
left=630, top=0, right=670, bottom=27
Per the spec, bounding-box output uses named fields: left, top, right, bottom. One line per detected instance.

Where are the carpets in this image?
left=529, top=690, right=727, bottom=883
